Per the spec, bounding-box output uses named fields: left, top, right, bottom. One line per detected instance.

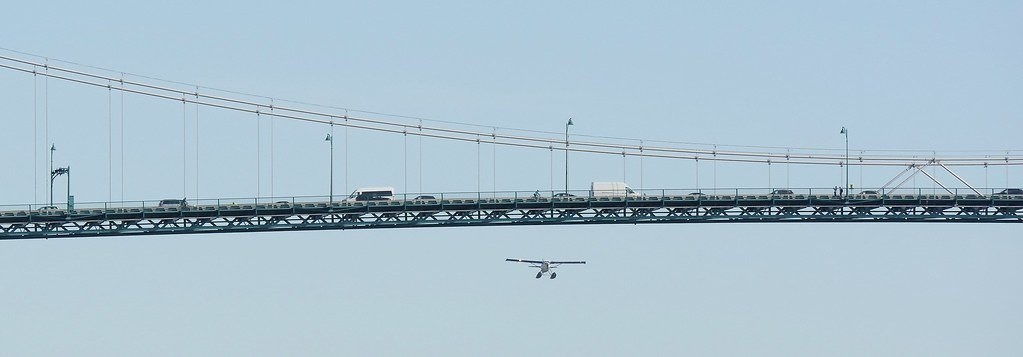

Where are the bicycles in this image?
left=531, top=195, right=545, bottom=202
left=178, top=203, right=190, bottom=211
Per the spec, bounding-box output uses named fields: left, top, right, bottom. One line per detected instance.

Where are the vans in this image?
left=159, top=199, right=188, bottom=205
left=591, top=182, right=637, bottom=196
left=342, top=187, right=395, bottom=200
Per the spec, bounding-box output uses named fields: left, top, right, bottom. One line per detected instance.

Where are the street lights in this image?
left=566, top=118, right=574, bottom=194
left=51, top=143, right=56, bottom=206
left=840, top=126, right=848, bottom=198
left=325, top=133, right=333, bottom=205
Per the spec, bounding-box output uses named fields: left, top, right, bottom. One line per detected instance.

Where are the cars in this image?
left=856, top=191, right=880, bottom=199
left=37, top=206, right=58, bottom=209
left=771, top=190, right=795, bottom=199
left=992, top=188, right=1023, bottom=198
left=414, top=196, right=435, bottom=199
left=685, top=193, right=706, bottom=200
left=550, top=193, right=574, bottom=201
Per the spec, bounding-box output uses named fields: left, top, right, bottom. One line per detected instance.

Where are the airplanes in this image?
left=505, top=258, right=587, bottom=279
